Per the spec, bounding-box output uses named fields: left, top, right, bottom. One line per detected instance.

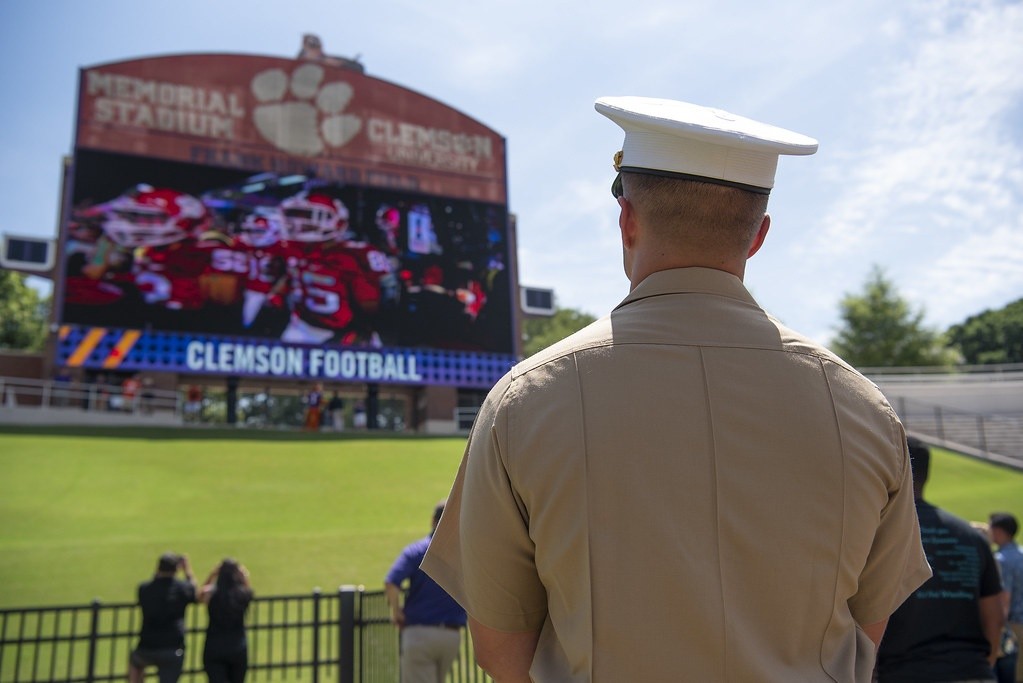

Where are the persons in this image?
left=198, top=559, right=254, bottom=683
left=418, top=96, right=934, bottom=683
left=870, top=435, right=1004, bottom=683
left=127, top=553, right=198, bottom=683
left=383, top=502, right=467, bottom=683
left=65, top=178, right=505, bottom=348
left=53, top=369, right=369, bottom=432
left=972, top=512, right=1023, bottom=683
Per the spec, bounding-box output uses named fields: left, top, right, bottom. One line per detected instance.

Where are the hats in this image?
left=595, top=94, right=818, bottom=199
left=159, top=555, right=177, bottom=573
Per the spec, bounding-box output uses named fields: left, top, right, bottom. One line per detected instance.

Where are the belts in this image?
left=409, top=619, right=462, bottom=630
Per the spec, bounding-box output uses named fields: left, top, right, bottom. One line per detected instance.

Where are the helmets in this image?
left=101, top=187, right=210, bottom=248
left=281, top=189, right=350, bottom=242
left=238, top=204, right=284, bottom=248
